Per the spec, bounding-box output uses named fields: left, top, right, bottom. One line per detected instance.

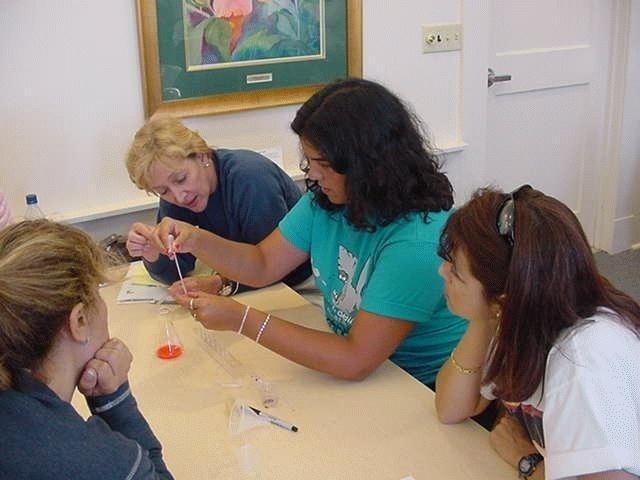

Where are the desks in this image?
left=69, top=252, right=523, bottom=480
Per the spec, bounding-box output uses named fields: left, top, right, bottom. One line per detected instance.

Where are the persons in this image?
left=434, top=185, right=640, bottom=480
left=1, top=219, right=173, bottom=480
left=149, top=77, right=469, bottom=392
left=126, top=116, right=324, bottom=315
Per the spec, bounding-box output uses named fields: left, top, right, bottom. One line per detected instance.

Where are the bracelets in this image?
left=238, top=304, right=252, bottom=333
left=255, top=314, right=272, bottom=344
left=451, top=348, right=483, bottom=375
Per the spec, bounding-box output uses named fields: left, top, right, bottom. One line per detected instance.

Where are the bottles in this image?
left=25, top=195, right=46, bottom=225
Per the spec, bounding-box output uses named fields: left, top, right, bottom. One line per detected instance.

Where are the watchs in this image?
left=215, top=273, right=232, bottom=297
left=517, top=454, right=544, bottom=480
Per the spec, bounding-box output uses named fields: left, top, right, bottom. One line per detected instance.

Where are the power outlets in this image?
left=421, top=25, right=460, bottom=53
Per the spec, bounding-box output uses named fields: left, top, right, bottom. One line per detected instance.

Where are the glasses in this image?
left=496, top=185, right=535, bottom=244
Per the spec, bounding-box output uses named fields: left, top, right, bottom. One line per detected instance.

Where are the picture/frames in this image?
left=135, top=0, right=363, bottom=119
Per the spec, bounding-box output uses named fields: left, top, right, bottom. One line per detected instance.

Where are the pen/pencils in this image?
left=240, top=405, right=299, bottom=433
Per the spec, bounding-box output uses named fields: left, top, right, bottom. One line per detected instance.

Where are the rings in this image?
left=190, top=297, right=193, bottom=309
left=193, top=313, right=197, bottom=320
